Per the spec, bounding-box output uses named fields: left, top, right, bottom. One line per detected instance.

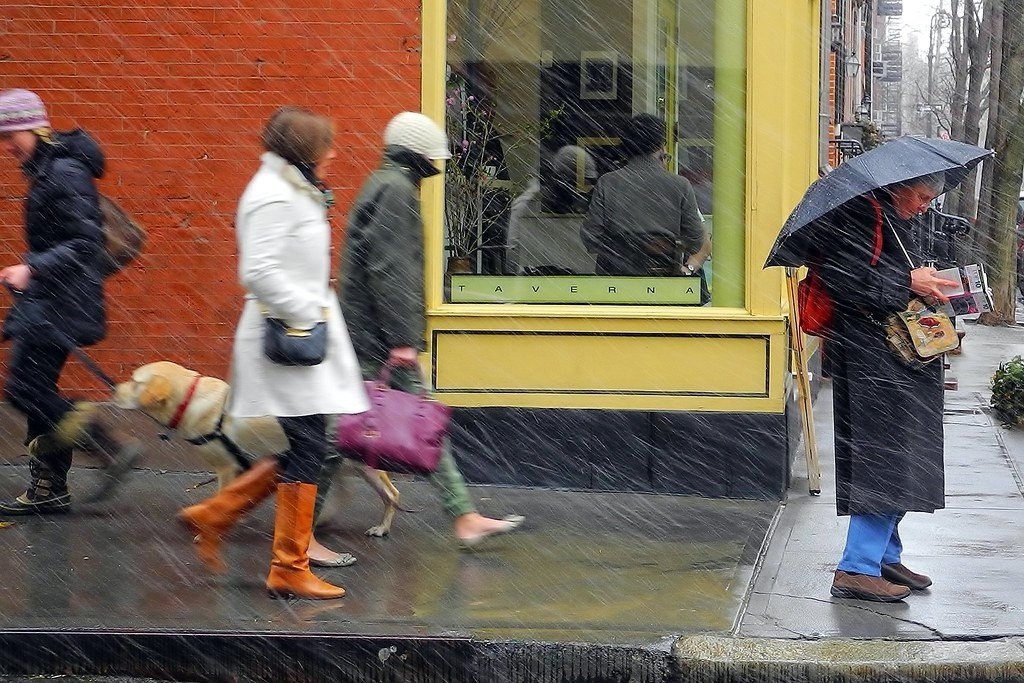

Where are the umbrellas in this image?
left=764, top=134, right=995, bottom=267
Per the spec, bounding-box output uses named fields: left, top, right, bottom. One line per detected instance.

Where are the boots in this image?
left=57, top=402, right=141, bottom=501
left=265, top=481, right=346, bottom=599
left=0, top=435, right=72, bottom=513
left=177, top=459, right=277, bottom=573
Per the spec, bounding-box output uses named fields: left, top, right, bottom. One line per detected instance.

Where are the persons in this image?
left=176, top=110, right=373, bottom=598
left=824, top=171, right=959, bottom=602
left=0, top=88, right=104, bottom=516
left=305, top=110, right=526, bottom=568
left=509, top=114, right=711, bottom=307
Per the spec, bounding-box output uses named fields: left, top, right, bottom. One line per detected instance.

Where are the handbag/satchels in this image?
left=97, top=192, right=146, bottom=278
left=264, top=318, right=328, bottom=367
left=881, top=296, right=959, bottom=372
left=336, top=356, right=450, bottom=476
left=798, top=195, right=883, bottom=336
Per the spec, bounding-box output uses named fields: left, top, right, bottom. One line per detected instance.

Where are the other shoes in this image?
left=882, top=563, right=932, bottom=589
left=831, top=570, right=911, bottom=602
left=457, top=514, right=525, bottom=552
left=308, top=552, right=356, bottom=566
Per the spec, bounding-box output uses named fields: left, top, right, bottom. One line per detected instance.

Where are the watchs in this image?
left=683, top=262, right=694, bottom=273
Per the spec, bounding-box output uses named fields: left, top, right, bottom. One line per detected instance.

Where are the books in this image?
left=932, top=262, right=995, bottom=317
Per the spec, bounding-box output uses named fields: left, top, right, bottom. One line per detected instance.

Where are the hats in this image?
left=623, top=113, right=665, bottom=153
left=556, top=146, right=597, bottom=178
left=0, top=88, right=51, bottom=131
left=384, top=112, right=453, bottom=160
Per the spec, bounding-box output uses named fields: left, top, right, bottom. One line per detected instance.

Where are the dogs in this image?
left=110, top=360, right=402, bottom=537
